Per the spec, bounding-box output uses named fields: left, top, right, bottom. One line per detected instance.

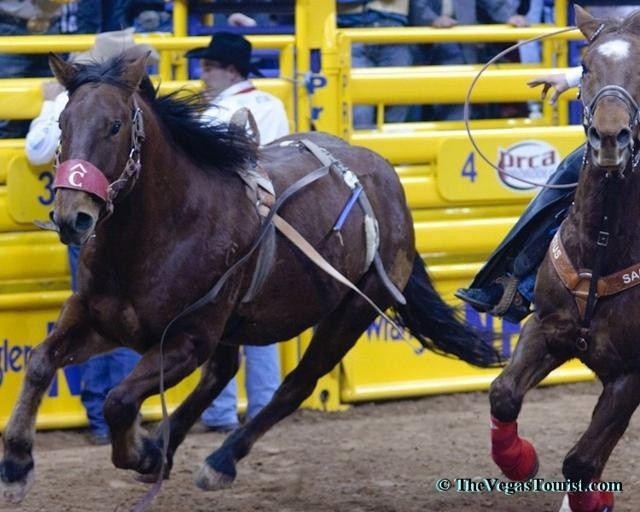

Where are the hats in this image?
left=182, top=29, right=265, bottom=80
left=67, top=29, right=162, bottom=70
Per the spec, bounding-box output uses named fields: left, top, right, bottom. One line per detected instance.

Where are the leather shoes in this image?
left=455, top=281, right=532, bottom=324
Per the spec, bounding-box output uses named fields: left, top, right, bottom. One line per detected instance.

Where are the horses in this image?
left=0, top=48, right=513, bottom=506
left=487, top=3, right=640, bottom=512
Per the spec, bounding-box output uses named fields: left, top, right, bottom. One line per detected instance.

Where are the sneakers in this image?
left=84, top=432, right=112, bottom=446
left=206, top=421, right=243, bottom=433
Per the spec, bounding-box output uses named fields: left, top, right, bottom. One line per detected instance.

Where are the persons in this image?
left=184, top=30, right=291, bottom=434
left=454, top=67, right=588, bottom=323
left=1, top=0, right=639, bottom=139
left=23, top=34, right=143, bottom=447
